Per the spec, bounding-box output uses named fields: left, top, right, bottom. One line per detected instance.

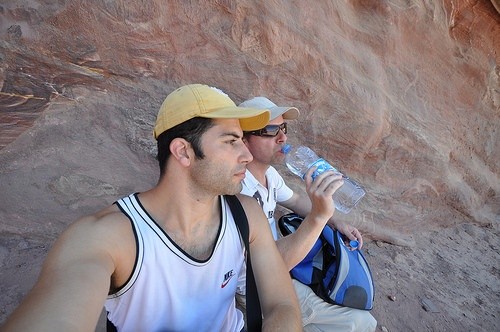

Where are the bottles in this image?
left=281, top=144, right=366, bottom=214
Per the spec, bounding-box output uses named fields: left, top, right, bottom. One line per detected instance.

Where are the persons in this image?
left=237, top=96, right=378, bottom=332
left=0, top=84, right=305, bottom=332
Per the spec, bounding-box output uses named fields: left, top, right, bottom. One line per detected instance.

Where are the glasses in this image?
left=247, top=123, right=288, bottom=138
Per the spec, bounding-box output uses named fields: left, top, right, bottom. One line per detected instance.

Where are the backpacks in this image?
left=279, top=212, right=376, bottom=312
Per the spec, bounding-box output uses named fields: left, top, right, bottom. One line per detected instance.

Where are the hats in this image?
left=236, top=96, right=301, bottom=121
left=152, top=84, right=269, bottom=140
